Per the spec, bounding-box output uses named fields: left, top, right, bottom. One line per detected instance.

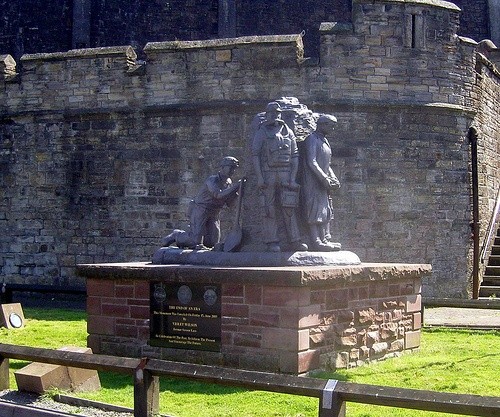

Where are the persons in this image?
left=252, top=102, right=309, bottom=251
left=301, top=113, right=343, bottom=251
left=159, top=153, right=244, bottom=248
